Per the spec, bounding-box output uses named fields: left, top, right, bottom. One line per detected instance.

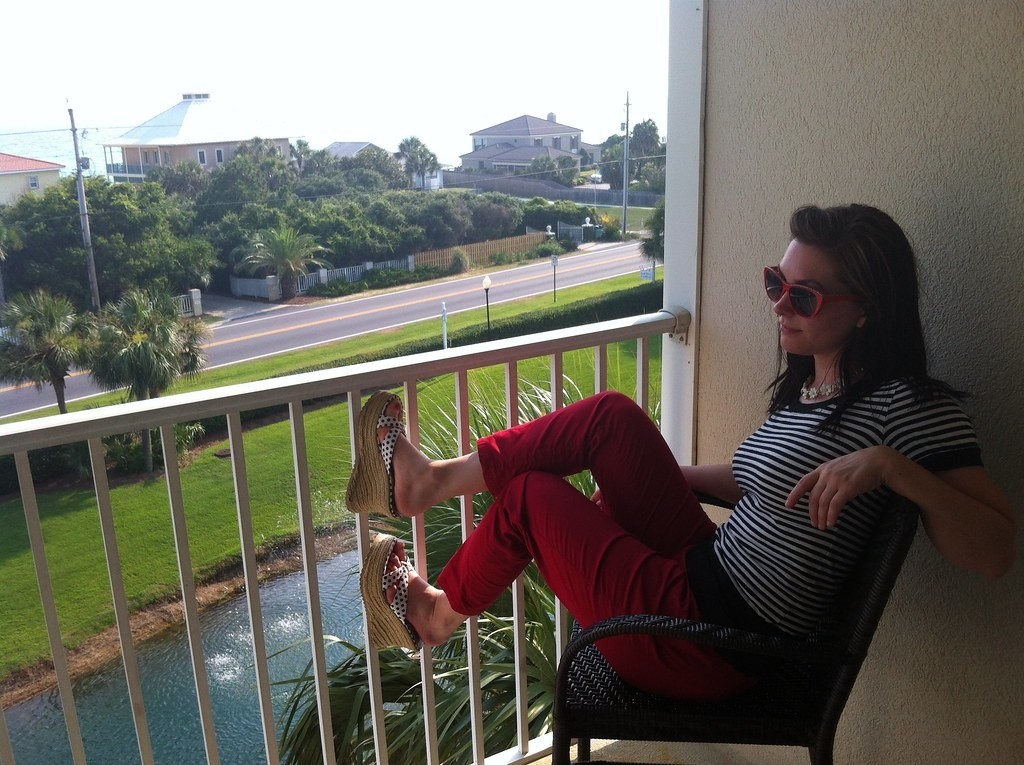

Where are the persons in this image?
left=345, top=204, right=1020, bottom=701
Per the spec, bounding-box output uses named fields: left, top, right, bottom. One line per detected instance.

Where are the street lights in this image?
left=482, top=275, right=492, bottom=341
left=592, top=163, right=599, bottom=213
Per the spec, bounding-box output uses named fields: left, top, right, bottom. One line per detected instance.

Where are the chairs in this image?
left=552, top=495, right=920, bottom=765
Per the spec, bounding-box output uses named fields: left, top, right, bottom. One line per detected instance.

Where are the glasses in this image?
left=764, top=265, right=866, bottom=320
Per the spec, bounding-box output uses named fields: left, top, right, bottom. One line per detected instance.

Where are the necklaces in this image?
left=801, top=365, right=864, bottom=399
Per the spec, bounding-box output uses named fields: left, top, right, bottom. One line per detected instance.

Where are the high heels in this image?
left=345, top=391, right=406, bottom=520
left=359, top=532, right=424, bottom=653
